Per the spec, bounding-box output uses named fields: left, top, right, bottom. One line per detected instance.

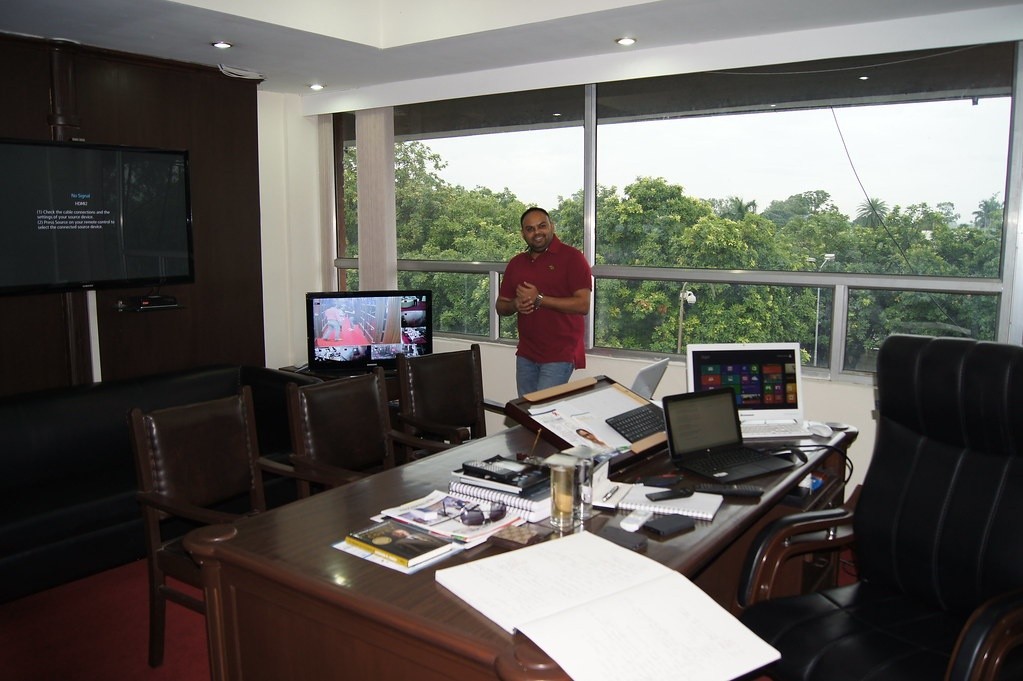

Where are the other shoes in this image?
left=335, top=338, right=343, bottom=341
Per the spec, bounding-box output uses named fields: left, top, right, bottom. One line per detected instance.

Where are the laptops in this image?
left=686, top=344, right=813, bottom=438
left=662, top=387, right=795, bottom=485
left=631, top=357, right=671, bottom=399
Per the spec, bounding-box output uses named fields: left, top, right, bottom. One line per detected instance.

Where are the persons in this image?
left=495, top=205, right=594, bottom=397
left=323, top=303, right=357, bottom=342
left=576, top=429, right=611, bottom=450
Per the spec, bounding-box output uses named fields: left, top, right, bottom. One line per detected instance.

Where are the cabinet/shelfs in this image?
left=278, top=365, right=404, bottom=465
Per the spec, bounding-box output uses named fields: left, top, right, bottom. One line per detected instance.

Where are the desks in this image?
left=179, top=417, right=857, bottom=681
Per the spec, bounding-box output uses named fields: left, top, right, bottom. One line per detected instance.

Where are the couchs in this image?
left=0, top=363, right=324, bottom=605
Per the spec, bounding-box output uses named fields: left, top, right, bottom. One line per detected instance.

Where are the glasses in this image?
left=430, top=495, right=507, bottom=527
left=582, top=433, right=591, bottom=438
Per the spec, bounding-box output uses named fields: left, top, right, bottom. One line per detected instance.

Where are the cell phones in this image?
left=645, top=488, right=693, bottom=501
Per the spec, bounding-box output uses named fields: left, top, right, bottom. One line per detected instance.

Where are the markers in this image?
left=603, top=486, right=618, bottom=502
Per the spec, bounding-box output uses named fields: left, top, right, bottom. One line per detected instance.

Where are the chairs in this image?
left=735, top=333, right=1023, bottom=681
left=131, top=386, right=353, bottom=669
left=288, top=366, right=459, bottom=499
left=395, top=344, right=506, bottom=460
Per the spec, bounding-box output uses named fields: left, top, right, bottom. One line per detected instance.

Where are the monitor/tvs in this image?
left=305, top=290, right=433, bottom=377
left=0, top=137, right=195, bottom=297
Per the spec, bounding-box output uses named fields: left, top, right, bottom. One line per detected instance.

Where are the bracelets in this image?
left=533, top=292, right=543, bottom=310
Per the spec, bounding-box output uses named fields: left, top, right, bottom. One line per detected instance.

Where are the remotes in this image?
left=694, top=484, right=764, bottom=498
left=462, top=460, right=518, bottom=480
left=619, top=509, right=653, bottom=533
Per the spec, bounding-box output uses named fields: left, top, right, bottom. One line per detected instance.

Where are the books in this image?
left=447, top=452, right=553, bottom=523
left=435, top=530, right=782, bottom=681
left=345, top=488, right=527, bottom=568
left=585, top=462, right=725, bottom=522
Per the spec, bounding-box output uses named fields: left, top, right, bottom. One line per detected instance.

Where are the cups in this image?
left=550, top=466, right=575, bottom=527
left=574, top=458, right=594, bottom=520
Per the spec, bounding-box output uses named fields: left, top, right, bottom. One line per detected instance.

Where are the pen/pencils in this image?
left=530, top=428, right=542, bottom=456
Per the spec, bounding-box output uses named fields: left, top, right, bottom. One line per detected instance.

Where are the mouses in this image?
left=808, top=424, right=833, bottom=437
left=769, top=447, right=808, bottom=466
